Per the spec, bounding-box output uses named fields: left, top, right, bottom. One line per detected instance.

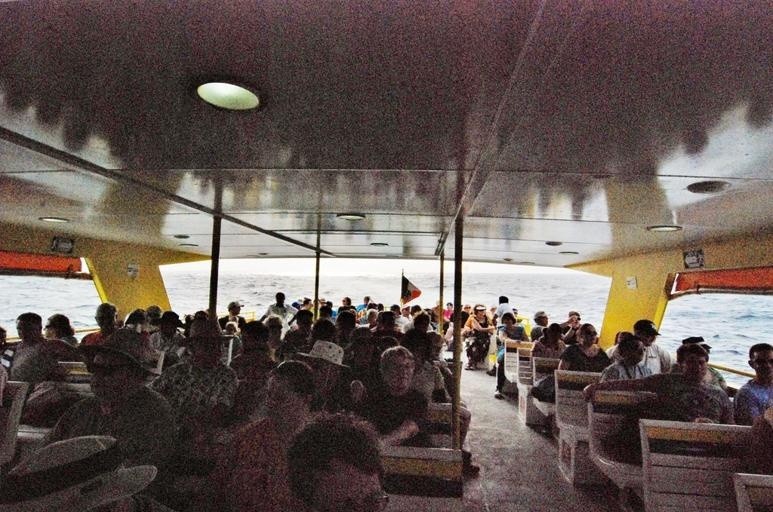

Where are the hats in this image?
left=75, top=329, right=162, bottom=376
left=263, top=315, right=282, bottom=327
left=227, top=302, right=244, bottom=312
left=176, top=319, right=234, bottom=345
left=683, top=337, right=712, bottom=349
left=0, top=435, right=158, bottom=511
left=296, top=340, right=352, bottom=372
left=292, top=302, right=303, bottom=307
left=534, top=311, right=552, bottom=319
left=475, top=306, right=486, bottom=310
left=634, top=320, right=660, bottom=335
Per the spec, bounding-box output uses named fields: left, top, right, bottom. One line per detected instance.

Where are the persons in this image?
left=633, top=319, right=675, bottom=373
left=669, top=336, right=729, bottom=392
left=735, top=344, right=771, bottom=426
left=530, top=323, right=566, bottom=368
left=494, top=313, right=529, bottom=399
left=558, top=322, right=610, bottom=390
left=2, top=293, right=480, bottom=510
left=461, top=296, right=517, bottom=370
left=597, top=331, right=653, bottom=382
left=582, top=343, right=734, bottom=459
left=531, top=310, right=582, bottom=344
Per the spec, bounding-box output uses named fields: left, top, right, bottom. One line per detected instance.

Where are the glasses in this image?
left=86, top=362, right=132, bottom=377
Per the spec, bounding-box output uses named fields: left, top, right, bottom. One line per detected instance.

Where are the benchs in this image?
left=554, top=371, right=773, bottom=511
left=505, top=341, right=561, bottom=426
left=380, top=363, right=467, bottom=510
left=2, top=333, right=89, bottom=451
